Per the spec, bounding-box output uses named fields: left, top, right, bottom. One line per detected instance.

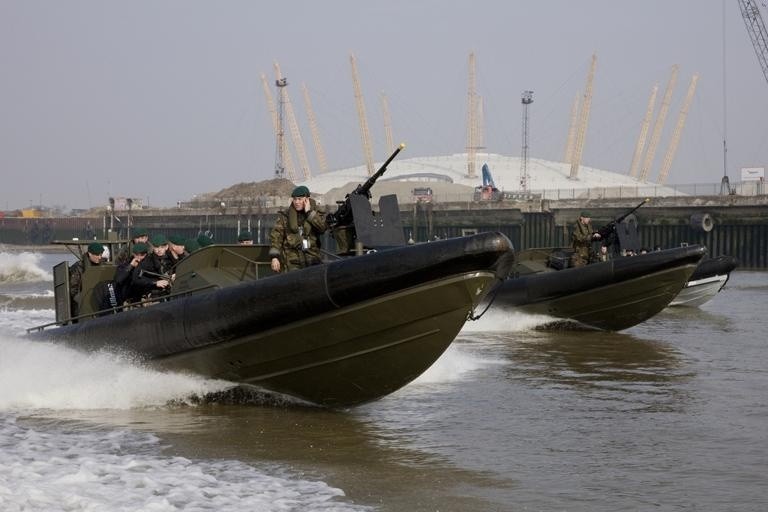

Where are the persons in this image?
left=570, top=211, right=601, bottom=267
left=268, top=186, right=327, bottom=274
left=237, top=231, right=253, bottom=245
left=335, top=189, right=378, bottom=257
left=69, top=242, right=108, bottom=324
left=599, top=242, right=662, bottom=262
left=115, top=228, right=214, bottom=312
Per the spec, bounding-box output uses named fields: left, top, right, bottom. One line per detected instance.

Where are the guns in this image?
left=334, top=145, right=404, bottom=225
left=592, top=198, right=650, bottom=241
left=139, top=269, right=175, bottom=285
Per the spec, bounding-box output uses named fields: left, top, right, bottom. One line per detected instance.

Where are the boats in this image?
left=482, top=197, right=738, bottom=332
left=16, top=142, right=516, bottom=412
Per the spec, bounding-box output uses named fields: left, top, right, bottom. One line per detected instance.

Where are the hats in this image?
left=130, top=227, right=149, bottom=238
left=87, top=242, right=105, bottom=255
left=132, top=234, right=215, bottom=254
left=237, top=231, right=253, bottom=242
left=581, top=210, right=592, bottom=219
left=291, top=186, right=310, bottom=198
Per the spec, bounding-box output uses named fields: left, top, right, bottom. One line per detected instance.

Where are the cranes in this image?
left=467, top=52, right=493, bottom=180
left=350, top=54, right=403, bottom=176
left=628, top=61, right=699, bottom=183
left=261, top=61, right=332, bottom=181
left=520, top=86, right=541, bottom=192
left=564, top=50, right=598, bottom=182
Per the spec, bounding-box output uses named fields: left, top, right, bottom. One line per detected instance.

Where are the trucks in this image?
left=473, top=186, right=501, bottom=201
left=412, top=186, right=433, bottom=205
